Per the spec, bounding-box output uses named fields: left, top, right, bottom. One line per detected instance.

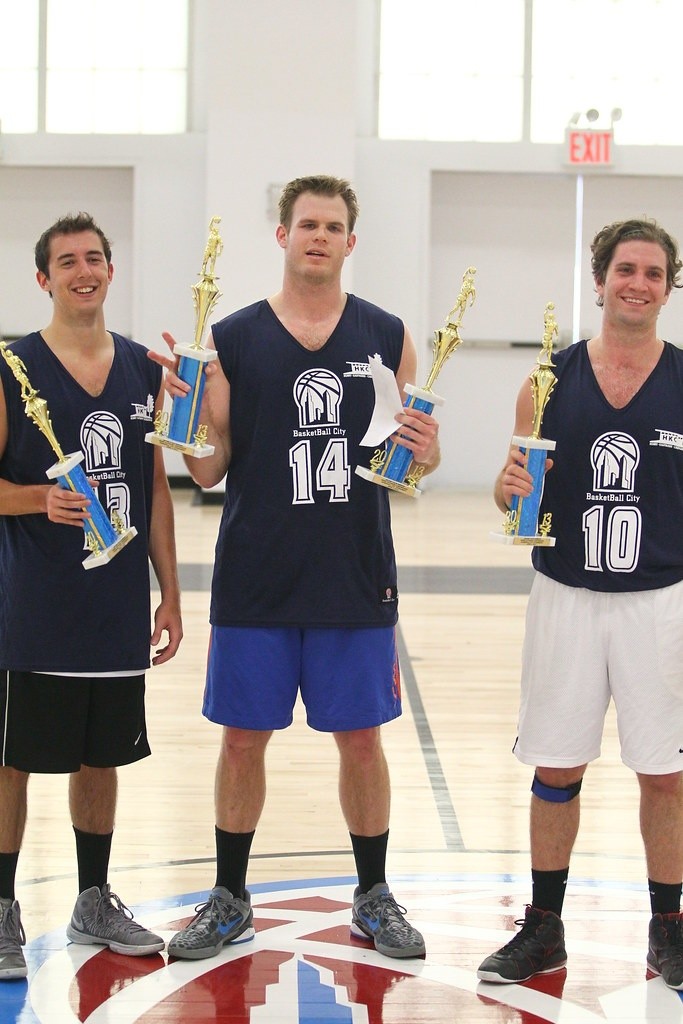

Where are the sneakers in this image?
left=477, top=907, right=568, bottom=983
left=0, top=898, right=27, bottom=979
left=67, top=883, right=166, bottom=955
left=350, top=883, right=425, bottom=957
left=646, top=912, right=683, bottom=991
left=167, top=886, right=255, bottom=959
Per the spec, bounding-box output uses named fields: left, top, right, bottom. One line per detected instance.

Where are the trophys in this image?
left=0, top=339, right=138, bottom=570
left=143, top=215, right=223, bottom=457
left=354, top=265, right=474, bottom=498
left=487, top=302, right=558, bottom=546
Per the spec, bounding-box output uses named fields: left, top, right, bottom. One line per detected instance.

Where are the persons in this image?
left=0, top=212, right=181, bottom=980
left=148, top=172, right=441, bottom=959
left=479, top=220, right=682, bottom=992
